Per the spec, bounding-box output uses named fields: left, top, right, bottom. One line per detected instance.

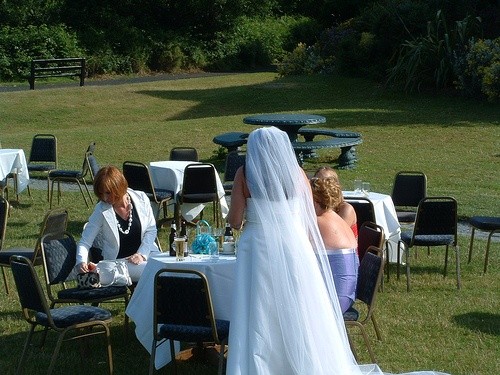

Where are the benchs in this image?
left=213, top=128, right=363, bottom=171
left=26, top=57, right=86, bottom=89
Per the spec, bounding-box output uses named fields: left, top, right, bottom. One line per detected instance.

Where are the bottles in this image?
left=169, top=220, right=178, bottom=256
left=178, top=221, right=188, bottom=257
left=223, top=219, right=234, bottom=242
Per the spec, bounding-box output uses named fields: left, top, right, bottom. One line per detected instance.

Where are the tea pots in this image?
left=191, top=220, right=217, bottom=254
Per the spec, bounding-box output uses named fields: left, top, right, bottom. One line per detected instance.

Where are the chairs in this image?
left=343, top=197, right=385, bottom=364
left=392, top=171, right=500, bottom=292
left=0, top=134, right=246, bottom=375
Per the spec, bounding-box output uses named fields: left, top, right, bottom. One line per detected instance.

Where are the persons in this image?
left=75, top=165, right=159, bottom=282
left=314, top=167, right=358, bottom=255
left=226, top=126, right=452, bottom=375
left=308, top=178, right=359, bottom=315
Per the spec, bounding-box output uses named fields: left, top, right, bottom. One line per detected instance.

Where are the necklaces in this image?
left=117, top=193, right=132, bottom=234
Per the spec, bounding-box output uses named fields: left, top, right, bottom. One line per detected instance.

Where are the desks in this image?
left=0, top=149, right=30, bottom=201
left=150, top=161, right=230, bottom=222
left=243, top=114, right=326, bottom=168
left=343, top=190, right=404, bottom=264
left=124, top=250, right=236, bottom=369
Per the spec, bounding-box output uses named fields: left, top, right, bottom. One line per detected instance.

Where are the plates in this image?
left=221, top=252, right=235, bottom=255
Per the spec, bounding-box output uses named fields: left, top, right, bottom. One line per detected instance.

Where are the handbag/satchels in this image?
left=192, top=221, right=218, bottom=254
left=76, top=272, right=101, bottom=289
left=96, top=260, right=132, bottom=287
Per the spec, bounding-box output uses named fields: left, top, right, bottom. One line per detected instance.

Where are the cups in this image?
left=198, top=225, right=223, bottom=251
left=354, top=179, right=362, bottom=195
left=174, top=236, right=185, bottom=261
left=222, top=242, right=235, bottom=252
left=208, top=242, right=220, bottom=263
left=361, top=182, right=370, bottom=198
